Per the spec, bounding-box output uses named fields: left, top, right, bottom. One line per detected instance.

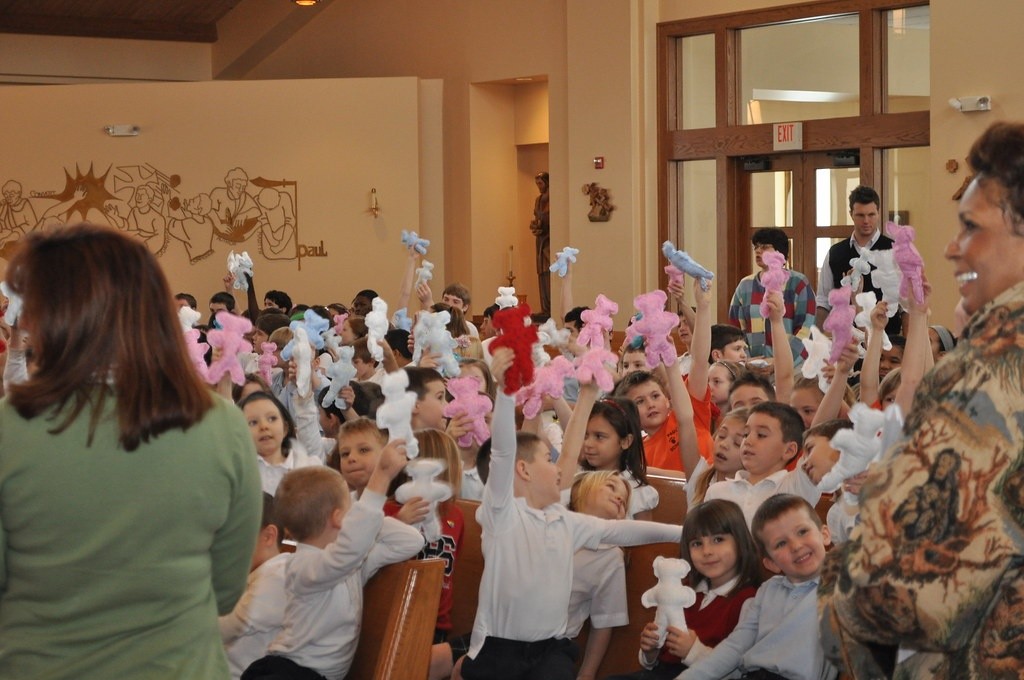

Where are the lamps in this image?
left=949, top=95, right=992, bottom=112
left=103, top=124, right=139, bottom=136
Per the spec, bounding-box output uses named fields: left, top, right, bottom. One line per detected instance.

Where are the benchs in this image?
left=282, top=471, right=835, bottom=680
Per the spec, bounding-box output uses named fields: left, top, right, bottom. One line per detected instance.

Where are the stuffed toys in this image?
left=0, top=226, right=925, bottom=649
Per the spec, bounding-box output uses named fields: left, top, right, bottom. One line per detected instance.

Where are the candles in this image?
left=509, top=245, right=513, bottom=276
left=372, top=188, right=376, bottom=208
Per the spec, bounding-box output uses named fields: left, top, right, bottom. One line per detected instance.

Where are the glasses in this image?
left=753, top=244, right=774, bottom=249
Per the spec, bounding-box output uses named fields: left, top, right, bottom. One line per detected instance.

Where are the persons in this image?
left=0, top=222, right=263, bottom=680
left=388, top=428, right=464, bottom=643
left=675, top=493, right=831, bottom=680
left=429, top=470, right=629, bottom=680
left=338, top=420, right=389, bottom=503
left=554, top=363, right=659, bottom=520
left=461, top=347, right=684, bottom=680
left=0, top=186, right=955, bottom=548
left=214, top=491, right=291, bottom=680
left=816, top=120, right=1024, bottom=680
left=239, top=440, right=424, bottom=680
left=529, top=172, right=551, bottom=323
left=645, top=337, right=754, bottom=514
left=697, top=337, right=859, bottom=533
left=238, top=391, right=323, bottom=496
left=638, top=499, right=770, bottom=669
